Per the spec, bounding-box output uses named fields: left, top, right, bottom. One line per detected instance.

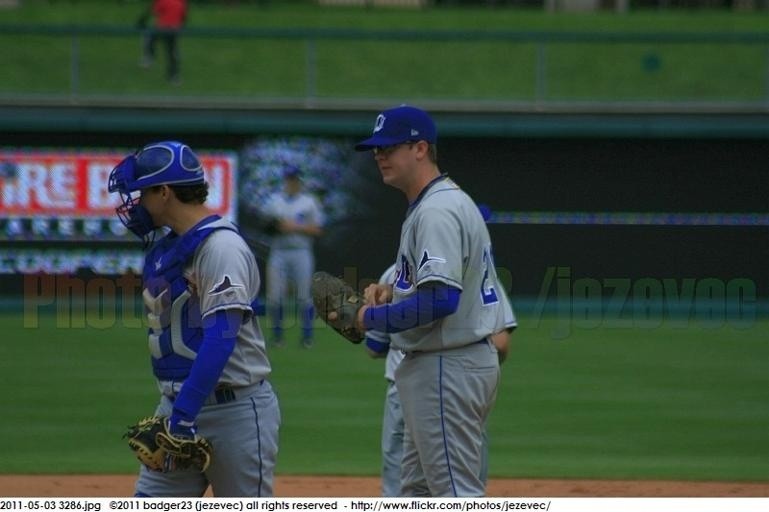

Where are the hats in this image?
left=355, top=106, right=437, bottom=152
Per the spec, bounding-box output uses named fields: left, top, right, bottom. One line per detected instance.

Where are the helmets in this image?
left=115, top=141, right=204, bottom=193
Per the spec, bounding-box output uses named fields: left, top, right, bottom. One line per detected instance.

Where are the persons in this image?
left=132, top=1, right=190, bottom=85
left=363, top=260, right=518, bottom=495
left=106, top=141, right=281, bottom=496
left=257, top=166, right=326, bottom=352
left=310, top=106, right=506, bottom=496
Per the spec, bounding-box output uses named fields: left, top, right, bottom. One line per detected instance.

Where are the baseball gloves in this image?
left=311, top=271, right=365, bottom=344
left=122, top=416, right=213, bottom=473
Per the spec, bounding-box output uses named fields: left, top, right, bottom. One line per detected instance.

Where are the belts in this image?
left=405, top=336, right=491, bottom=356
left=167, top=378, right=264, bottom=408
